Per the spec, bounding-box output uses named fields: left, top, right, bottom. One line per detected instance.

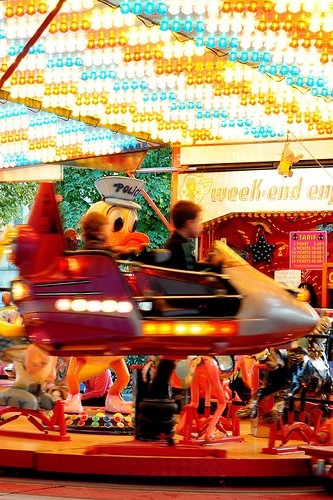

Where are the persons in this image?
left=80, top=369, right=114, bottom=406
left=83, top=211, right=127, bottom=253
left=156, top=200, right=224, bottom=275
left=298, top=283, right=322, bottom=308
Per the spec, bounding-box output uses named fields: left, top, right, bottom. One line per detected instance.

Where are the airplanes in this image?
left=1, top=183, right=318, bottom=358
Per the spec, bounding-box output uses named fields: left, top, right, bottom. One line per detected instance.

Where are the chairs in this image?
left=139, top=249, right=226, bottom=317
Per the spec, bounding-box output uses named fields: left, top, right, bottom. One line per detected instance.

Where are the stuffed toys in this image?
left=61, top=176, right=149, bottom=414
left=277, top=140, right=304, bottom=178
